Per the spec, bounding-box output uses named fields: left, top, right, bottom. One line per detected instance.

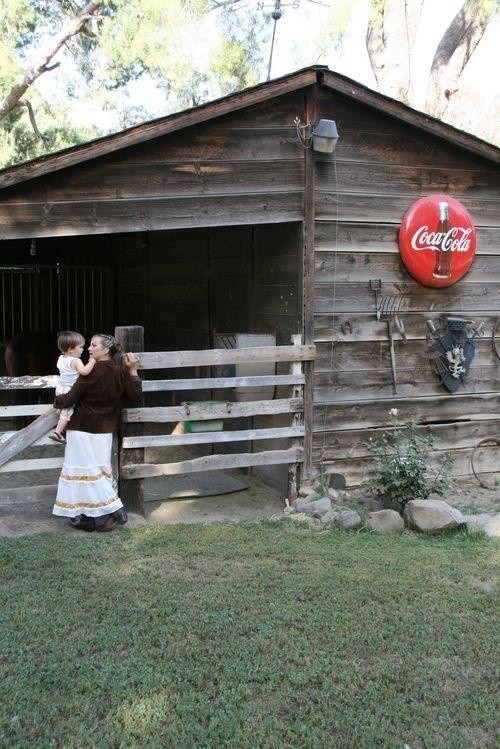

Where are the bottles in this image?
left=433, top=202, right=452, bottom=279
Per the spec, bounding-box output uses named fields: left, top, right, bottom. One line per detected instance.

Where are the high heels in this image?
left=96, top=508, right=127, bottom=531
left=68, top=516, right=95, bottom=532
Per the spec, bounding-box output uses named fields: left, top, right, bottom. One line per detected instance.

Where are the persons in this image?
left=53, top=333, right=142, bottom=533
left=49, top=331, right=96, bottom=444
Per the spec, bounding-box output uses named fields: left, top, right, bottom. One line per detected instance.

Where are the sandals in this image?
left=48, top=428, right=67, bottom=444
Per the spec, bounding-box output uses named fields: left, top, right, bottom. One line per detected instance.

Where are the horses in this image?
left=3, top=329, right=59, bottom=431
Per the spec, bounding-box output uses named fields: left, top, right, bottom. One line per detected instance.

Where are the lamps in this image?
left=294, top=116, right=339, bottom=154
